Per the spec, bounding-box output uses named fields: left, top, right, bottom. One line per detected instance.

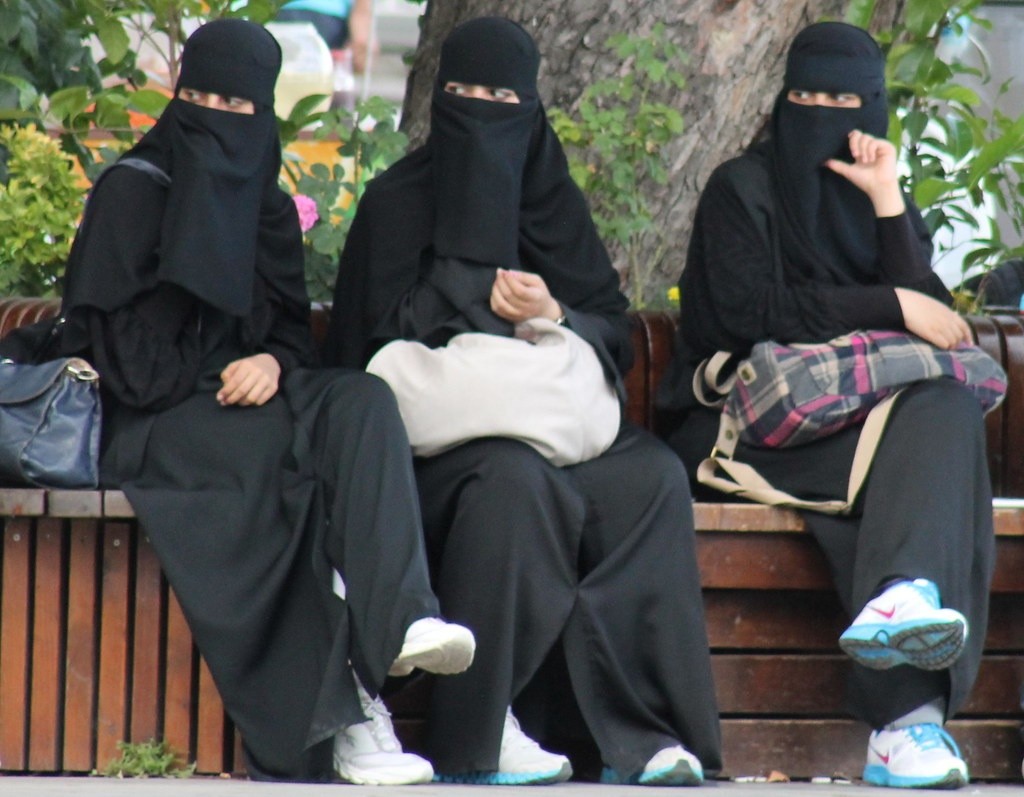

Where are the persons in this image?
left=321, top=16, right=724, bottom=785
left=274, top=0, right=380, bottom=74
left=63, top=17, right=478, bottom=785
left=656, top=20, right=996, bottom=787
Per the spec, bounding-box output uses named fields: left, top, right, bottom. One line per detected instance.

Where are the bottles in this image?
left=324, top=49, right=358, bottom=129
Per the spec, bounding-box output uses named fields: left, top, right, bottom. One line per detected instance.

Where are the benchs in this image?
left=1, top=488, right=1024, bottom=780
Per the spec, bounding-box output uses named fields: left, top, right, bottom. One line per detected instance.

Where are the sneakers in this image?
left=839, top=578, right=969, bottom=670
left=432, top=705, right=573, bottom=785
left=333, top=668, right=434, bottom=786
left=601, top=744, right=704, bottom=787
left=863, top=723, right=969, bottom=790
left=388, top=617, right=476, bottom=676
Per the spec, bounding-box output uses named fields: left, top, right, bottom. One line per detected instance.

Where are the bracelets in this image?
left=557, top=316, right=565, bottom=324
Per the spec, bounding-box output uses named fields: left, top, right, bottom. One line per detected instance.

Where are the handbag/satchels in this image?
left=0, top=357, right=103, bottom=490
left=692, top=329, right=1008, bottom=514
left=366, top=317, right=621, bottom=467
